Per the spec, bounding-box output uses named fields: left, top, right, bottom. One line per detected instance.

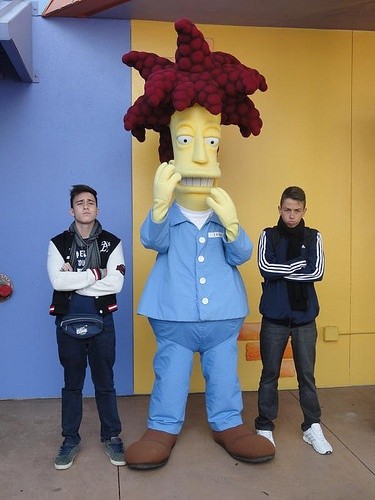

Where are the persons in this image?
left=47, top=185, right=126, bottom=469
left=255, top=187, right=333, bottom=455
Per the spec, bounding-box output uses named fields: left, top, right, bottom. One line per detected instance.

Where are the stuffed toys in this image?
left=122, top=20, right=275, bottom=467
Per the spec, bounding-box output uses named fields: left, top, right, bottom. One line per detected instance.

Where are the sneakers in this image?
left=302, top=423, right=334, bottom=456
left=54, top=436, right=80, bottom=470
left=256, top=429, right=276, bottom=450
left=104, top=436, right=126, bottom=466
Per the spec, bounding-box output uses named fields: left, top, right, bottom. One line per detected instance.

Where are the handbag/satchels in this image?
left=60, top=314, right=104, bottom=339
